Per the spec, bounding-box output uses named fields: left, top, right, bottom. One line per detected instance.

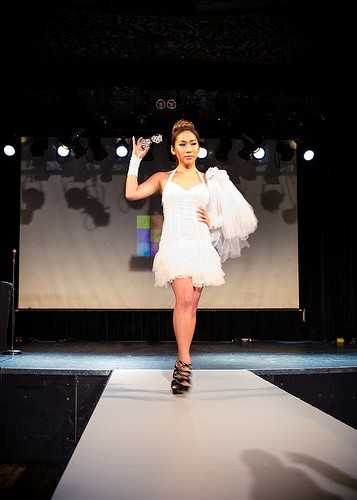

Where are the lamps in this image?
left=56, top=135, right=271, bottom=161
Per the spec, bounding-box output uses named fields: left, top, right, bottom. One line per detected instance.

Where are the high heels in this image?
left=170, top=358, right=193, bottom=395
